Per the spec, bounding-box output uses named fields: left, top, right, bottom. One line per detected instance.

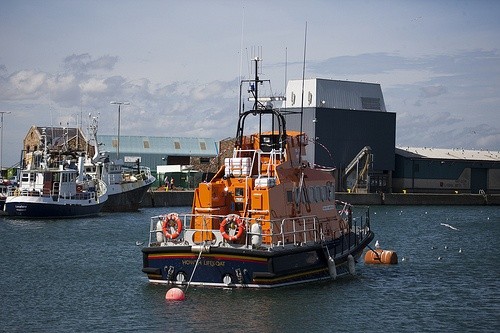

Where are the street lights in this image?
left=0, top=111, right=12, bottom=169
left=110, top=100, right=131, bottom=160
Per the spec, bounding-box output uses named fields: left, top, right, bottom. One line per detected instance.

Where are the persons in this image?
left=170, top=177, right=174, bottom=191
left=165, top=175, right=169, bottom=191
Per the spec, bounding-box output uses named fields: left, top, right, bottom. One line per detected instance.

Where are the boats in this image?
left=0, top=110, right=158, bottom=218
left=140, top=19, right=376, bottom=288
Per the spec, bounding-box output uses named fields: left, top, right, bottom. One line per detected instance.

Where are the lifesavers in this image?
left=161, top=214, right=183, bottom=240
left=219, top=213, right=244, bottom=242
left=76, top=185, right=83, bottom=192
